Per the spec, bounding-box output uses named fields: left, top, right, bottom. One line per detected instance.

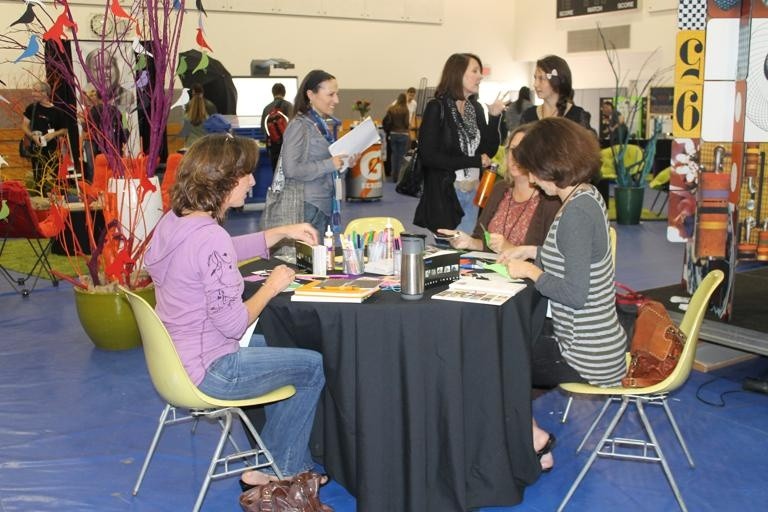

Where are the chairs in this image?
left=555, top=268, right=724, bottom=512
left=118, top=286, right=297, bottom=512
left=0, top=180, right=70, bottom=297
left=649, top=166, right=671, bottom=217
left=597, top=144, right=643, bottom=179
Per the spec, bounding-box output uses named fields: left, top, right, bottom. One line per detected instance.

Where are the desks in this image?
left=233, top=256, right=546, bottom=510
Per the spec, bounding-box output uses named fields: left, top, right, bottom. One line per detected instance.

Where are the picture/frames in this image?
left=70, top=40, right=143, bottom=162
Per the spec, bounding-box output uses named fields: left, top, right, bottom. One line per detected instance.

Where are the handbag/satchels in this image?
left=239, top=471, right=338, bottom=511
left=393, top=97, right=446, bottom=198
left=257, top=119, right=311, bottom=231
left=615, top=279, right=685, bottom=388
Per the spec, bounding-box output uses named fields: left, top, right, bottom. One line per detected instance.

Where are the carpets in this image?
left=608, top=195, right=667, bottom=222
left=0, top=237, right=102, bottom=284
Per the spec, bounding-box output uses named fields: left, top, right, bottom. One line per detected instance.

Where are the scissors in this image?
left=480, top=222, right=490, bottom=246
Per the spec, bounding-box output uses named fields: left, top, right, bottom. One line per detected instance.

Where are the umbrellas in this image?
left=176, top=49, right=238, bottom=115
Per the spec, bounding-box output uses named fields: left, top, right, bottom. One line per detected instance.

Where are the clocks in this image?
left=91, top=14, right=113, bottom=37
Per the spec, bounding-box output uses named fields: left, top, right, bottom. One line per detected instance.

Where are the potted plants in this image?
left=0, top=0, right=214, bottom=353
left=594, top=22, right=675, bottom=226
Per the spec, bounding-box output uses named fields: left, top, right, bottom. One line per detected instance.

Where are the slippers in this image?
left=237, top=472, right=259, bottom=494
left=535, top=435, right=557, bottom=462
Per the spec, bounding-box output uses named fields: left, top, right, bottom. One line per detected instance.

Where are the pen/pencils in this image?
left=460, top=264, right=483, bottom=270
left=368, top=230, right=387, bottom=262
left=339, top=230, right=370, bottom=274
left=392, top=237, right=402, bottom=251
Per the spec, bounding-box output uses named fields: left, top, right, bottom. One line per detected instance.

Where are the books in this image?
left=290, top=279, right=383, bottom=303
left=430, top=276, right=528, bottom=306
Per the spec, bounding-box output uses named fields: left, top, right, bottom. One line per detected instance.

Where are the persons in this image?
left=185, top=84, right=217, bottom=126
left=521, top=56, right=590, bottom=129
left=382, top=94, right=411, bottom=184
left=22, top=81, right=72, bottom=198
left=282, top=69, right=361, bottom=246
left=436, top=122, right=562, bottom=256
left=85, top=48, right=134, bottom=104
left=261, top=84, right=294, bottom=174
left=505, top=87, right=533, bottom=132
left=142, top=133, right=331, bottom=493
left=413, top=53, right=511, bottom=246
left=79, top=88, right=122, bottom=183
left=391, top=86, right=416, bottom=127
left=604, top=102, right=628, bottom=147
left=495, top=118, right=626, bottom=473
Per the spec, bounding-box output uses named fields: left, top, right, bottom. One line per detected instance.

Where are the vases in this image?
left=360, top=117, right=365, bottom=123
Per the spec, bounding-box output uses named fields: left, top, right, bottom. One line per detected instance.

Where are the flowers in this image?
left=352, top=100, right=370, bottom=117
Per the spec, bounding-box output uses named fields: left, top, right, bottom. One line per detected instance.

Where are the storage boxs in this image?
left=50, top=202, right=104, bottom=256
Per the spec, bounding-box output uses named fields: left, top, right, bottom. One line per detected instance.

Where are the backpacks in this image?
left=264, top=101, right=288, bottom=145
